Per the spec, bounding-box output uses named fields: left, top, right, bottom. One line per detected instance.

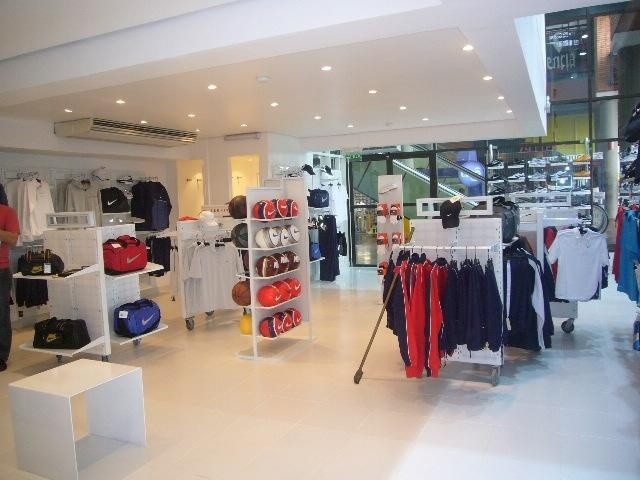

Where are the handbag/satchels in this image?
left=469, top=196, right=520, bottom=243
left=100, top=187, right=129, bottom=212
left=114, top=299, right=161, bottom=337
left=307, top=188, right=329, bottom=208
left=32, top=317, right=91, bottom=349
left=103, top=235, right=147, bottom=275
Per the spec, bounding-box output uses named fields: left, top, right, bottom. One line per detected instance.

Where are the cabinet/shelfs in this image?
left=13, top=223, right=167, bottom=362
left=487, top=161, right=591, bottom=224
left=235, top=177, right=308, bottom=361
left=309, top=206, right=331, bottom=264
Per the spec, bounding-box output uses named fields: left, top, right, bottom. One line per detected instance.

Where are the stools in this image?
left=8, top=358, right=146, bottom=480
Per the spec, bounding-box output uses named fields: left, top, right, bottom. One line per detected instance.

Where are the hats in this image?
left=200, top=211, right=218, bottom=226
left=440, top=199, right=462, bottom=229
left=304, top=165, right=316, bottom=175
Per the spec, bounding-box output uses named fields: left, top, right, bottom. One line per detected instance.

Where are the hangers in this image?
left=194, top=230, right=232, bottom=247
left=320, top=178, right=341, bottom=187
left=8, top=174, right=159, bottom=186
left=403, top=220, right=590, bottom=270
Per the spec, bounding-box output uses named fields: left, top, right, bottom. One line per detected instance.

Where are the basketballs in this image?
left=229, top=195, right=247, bottom=219
left=232, top=280, right=251, bottom=306
left=231, top=223, right=248, bottom=248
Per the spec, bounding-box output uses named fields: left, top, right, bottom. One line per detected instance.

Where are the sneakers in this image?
left=487, top=170, right=591, bottom=182
left=487, top=155, right=592, bottom=167
left=488, top=185, right=591, bottom=195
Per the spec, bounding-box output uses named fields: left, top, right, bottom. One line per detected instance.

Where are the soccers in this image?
left=240, top=314, right=253, bottom=335
left=255, top=251, right=300, bottom=277
left=252, top=199, right=298, bottom=223
left=257, top=278, right=301, bottom=307
left=255, top=225, right=300, bottom=248
left=259, top=308, right=302, bottom=338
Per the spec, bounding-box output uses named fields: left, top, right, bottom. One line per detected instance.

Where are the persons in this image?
left=1, top=183, right=21, bottom=374
